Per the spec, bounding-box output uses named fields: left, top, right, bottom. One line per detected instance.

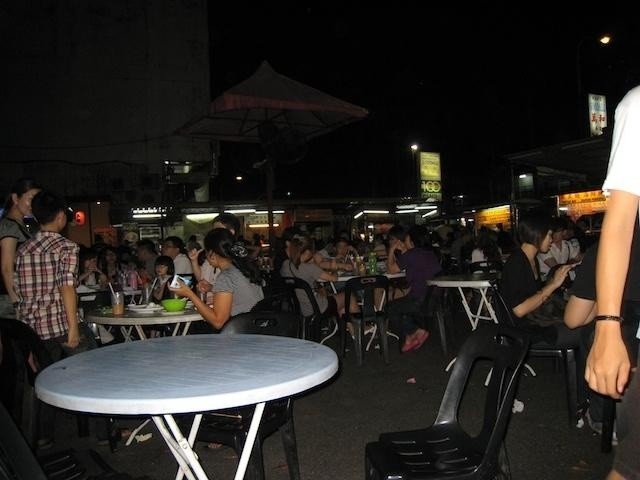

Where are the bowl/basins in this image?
left=162, top=299, right=187, bottom=312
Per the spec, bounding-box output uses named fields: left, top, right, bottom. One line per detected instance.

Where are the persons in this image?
left=316, top=229, right=389, bottom=272
left=11, top=188, right=132, bottom=451
left=1, top=176, right=41, bottom=410
left=584, top=85, right=640, bottom=479
left=537, top=217, right=584, bottom=282
left=150, top=256, right=176, bottom=305
left=189, top=213, right=241, bottom=303
left=462, top=235, right=503, bottom=329
left=432, top=225, right=473, bottom=276
left=165, top=228, right=264, bottom=334
left=237, top=233, right=264, bottom=248
left=279, top=234, right=391, bottom=340
left=389, top=225, right=412, bottom=300
left=273, top=227, right=321, bottom=270
left=137, top=238, right=158, bottom=283
left=385, top=225, right=447, bottom=352
left=500, top=211, right=595, bottom=349
left=563, top=212, right=640, bottom=445
left=162, top=236, right=194, bottom=300
left=186, top=235, right=200, bottom=253
left=77, top=236, right=138, bottom=288
left=563, top=220, right=587, bottom=254
left=496, top=223, right=517, bottom=249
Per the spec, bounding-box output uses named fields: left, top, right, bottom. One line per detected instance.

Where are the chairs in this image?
left=0, top=317, right=116, bottom=453
left=1, top=404, right=118, bottom=480
left=163, top=310, right=306, bottom=480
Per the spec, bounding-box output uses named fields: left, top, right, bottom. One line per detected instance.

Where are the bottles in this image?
left=369, top=252, right=376, bottom=275
left=355, top=254, right=361, bottom=265
left=359, top=263, right=367, bottom=277
left=128, top=271, right=138, bottom=290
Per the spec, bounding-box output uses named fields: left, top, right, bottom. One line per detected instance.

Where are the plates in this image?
left=128, top=306, right=164, bottom=314
left=128, top=304, right=146, bottom=308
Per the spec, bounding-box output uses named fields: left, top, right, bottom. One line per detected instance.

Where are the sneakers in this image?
left=587, top=407, right=619, bottom=446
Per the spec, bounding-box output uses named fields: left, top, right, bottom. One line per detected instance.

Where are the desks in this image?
left=34, top=333, right=339, bottom=480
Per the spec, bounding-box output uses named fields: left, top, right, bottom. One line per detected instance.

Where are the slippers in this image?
left=401, top=338, right=419, bottom=352
left=415, top=329, right=430, bottom=349
left=37, top=436, right=55, bottom=450
left=97, top=429, right=131, bottom=446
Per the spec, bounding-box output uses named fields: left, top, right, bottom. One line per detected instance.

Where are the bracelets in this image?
left=592, top=314, right=624, bottom=326
left=12, top=300, right=19, bottom=309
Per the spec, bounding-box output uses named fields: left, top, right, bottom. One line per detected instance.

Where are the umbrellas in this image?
left=173, top=60, right=370, bottom=252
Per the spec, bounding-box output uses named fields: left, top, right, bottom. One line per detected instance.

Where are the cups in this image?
left=110, top=291, right=125, bottom=317
